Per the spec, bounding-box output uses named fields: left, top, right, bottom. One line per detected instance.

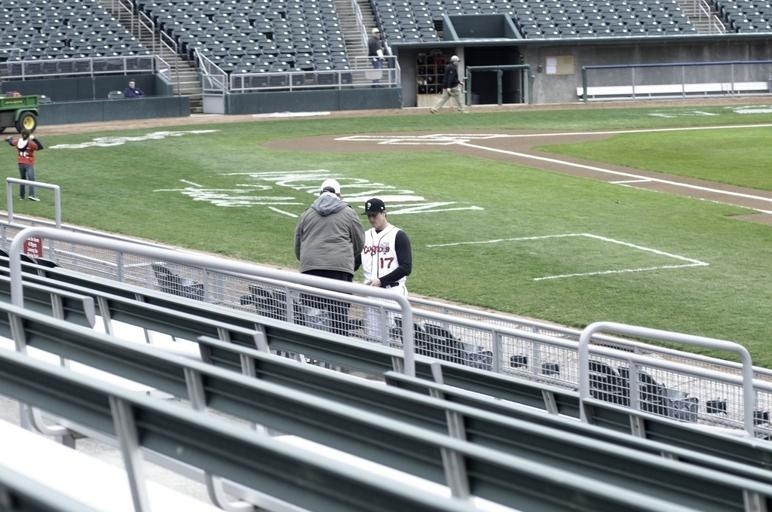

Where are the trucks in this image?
left=0, top=93, right=42, bottom=137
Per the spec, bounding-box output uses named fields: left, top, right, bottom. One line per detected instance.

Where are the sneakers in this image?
left=457, top=109, right=469, bottom=114
left=431, top=109, right=439, bottom=116
left=28, top=196, right=41, bottom=201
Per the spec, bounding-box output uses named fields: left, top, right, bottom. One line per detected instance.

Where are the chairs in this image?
left=1, top=0, right=772, bottom=93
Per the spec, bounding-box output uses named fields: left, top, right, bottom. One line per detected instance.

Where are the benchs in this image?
left=577, top=80, right=769, bottom=102
left=1, top=252, right=772, bottom=511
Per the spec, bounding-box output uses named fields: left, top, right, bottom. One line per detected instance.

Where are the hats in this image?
left=371, top=27, right=380, bottom=33
left=320, top=178, right=341, bottom=195
left=450, top=55, right=460, bottom=62
left=360, top=198, right=385, bottom=215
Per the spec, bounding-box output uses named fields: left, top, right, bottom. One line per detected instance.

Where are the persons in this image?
left=5, top=128, right=44, bottom=202
left=428, top=54, right=470, bottom=114
left=360, top=197, right=414, bottom=316
left=121, top=80, right=145, bottom=99
left=293, top=177, right=366, bottom=336
left=366, top=26, right=389, bottom=89
